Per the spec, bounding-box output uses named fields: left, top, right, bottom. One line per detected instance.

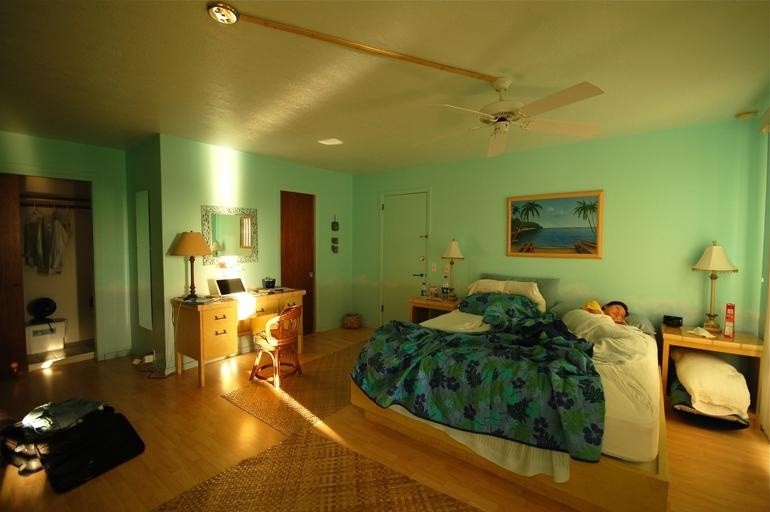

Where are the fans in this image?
left=27, top=298, right=58, bottom=333
left=410, top=78, right=604, bottom=159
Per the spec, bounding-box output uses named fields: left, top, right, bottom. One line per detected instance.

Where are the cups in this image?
left=265, top=279, right=275, bottom=288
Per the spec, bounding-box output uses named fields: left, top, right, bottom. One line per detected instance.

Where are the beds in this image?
left=351, top=275, right=670, bottom=512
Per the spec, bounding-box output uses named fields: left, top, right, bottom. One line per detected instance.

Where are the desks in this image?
left=170, top=286, right=306, bottom=387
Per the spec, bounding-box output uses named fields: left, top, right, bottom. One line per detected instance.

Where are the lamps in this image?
left=441, top=238, right=464, bottom=300
left=691, top=239, right=739, bottom=334
left=171, top=230, right=212, bottom=301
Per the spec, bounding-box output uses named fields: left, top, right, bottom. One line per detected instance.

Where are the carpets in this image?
left=220, top=339, right=372, bottom=437
left=149, top=429, right=485, bottom=512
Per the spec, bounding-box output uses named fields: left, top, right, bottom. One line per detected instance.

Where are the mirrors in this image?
left=134, top=189, right=153, bottom=330
left=201, top=205, right=259, bottom=266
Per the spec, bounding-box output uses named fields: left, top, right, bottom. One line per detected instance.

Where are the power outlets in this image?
left=151, top=349, right=155, bottom=360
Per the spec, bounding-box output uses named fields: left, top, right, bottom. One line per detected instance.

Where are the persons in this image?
left=580, top=300, right=631, bottom=326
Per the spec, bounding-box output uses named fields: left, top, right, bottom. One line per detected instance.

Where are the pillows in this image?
left=481, top=273, right=560, bottom=312
left=624, top=312, right=657, bottom=336
left=468, top=279, right=547, bottom=315
left=460, top=292, right=537, bottom=316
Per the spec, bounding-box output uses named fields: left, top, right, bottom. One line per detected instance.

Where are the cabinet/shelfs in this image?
left=25, top=318, right=68, bottom=355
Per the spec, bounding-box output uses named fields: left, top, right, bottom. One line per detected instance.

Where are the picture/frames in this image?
left=506, top=190, right=603, bottom=260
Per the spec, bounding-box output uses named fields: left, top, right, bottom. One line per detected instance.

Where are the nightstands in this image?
left=660, top=323, right=765, bottom=428
left=408, top=296, right=460, bottom=324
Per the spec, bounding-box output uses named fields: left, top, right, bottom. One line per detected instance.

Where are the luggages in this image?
left=0, top=397, right=145, bottom=494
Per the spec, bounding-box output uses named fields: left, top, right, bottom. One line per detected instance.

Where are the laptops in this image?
left=215, top=277, right=261, bottom=300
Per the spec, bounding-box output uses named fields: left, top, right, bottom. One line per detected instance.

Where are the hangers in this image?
left=25, top=200, right=70, bottom=224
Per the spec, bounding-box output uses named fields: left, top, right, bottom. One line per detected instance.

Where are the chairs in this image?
left=249, top=305, right=302, bottom=387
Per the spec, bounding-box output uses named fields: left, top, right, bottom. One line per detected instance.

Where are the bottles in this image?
left=440, top=275, right=450, bottom=304
left=421, top=281, right=427, bottom=301
